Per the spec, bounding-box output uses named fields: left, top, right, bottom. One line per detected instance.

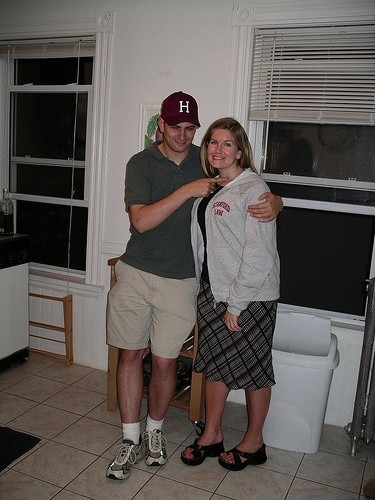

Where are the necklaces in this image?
left=210, top=173, right=237, bottom=194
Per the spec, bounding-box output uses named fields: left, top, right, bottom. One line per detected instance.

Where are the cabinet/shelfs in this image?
left=0, top=233, right=30, bottom=360
left=107, top=257, right=206, bottom=422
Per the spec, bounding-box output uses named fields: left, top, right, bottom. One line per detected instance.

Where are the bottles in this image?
left=0, top=188, right=14, bottom=236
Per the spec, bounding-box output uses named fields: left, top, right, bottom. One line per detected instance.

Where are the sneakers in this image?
left=142, top=424, right=168, bottom=466
left=106, top=433, right=145, bottom=480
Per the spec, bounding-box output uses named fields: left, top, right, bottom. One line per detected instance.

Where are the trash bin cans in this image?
left=262, top=311, right=341, bottom=453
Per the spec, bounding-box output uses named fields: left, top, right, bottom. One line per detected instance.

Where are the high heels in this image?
left=181, top=437, right=224, bottom=466
left=217, top=442, right=267, bottom=471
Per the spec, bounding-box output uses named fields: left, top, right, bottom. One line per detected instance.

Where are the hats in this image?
left=160, top=91, right=201, bottom=127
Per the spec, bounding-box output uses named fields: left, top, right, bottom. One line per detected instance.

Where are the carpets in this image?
left=0, top=427, right=42, bottom=476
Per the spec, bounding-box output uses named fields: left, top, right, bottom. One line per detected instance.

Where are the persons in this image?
left=180, top=117, right=280, bottom=472
left=105, top=91, right=284, bottom=481
